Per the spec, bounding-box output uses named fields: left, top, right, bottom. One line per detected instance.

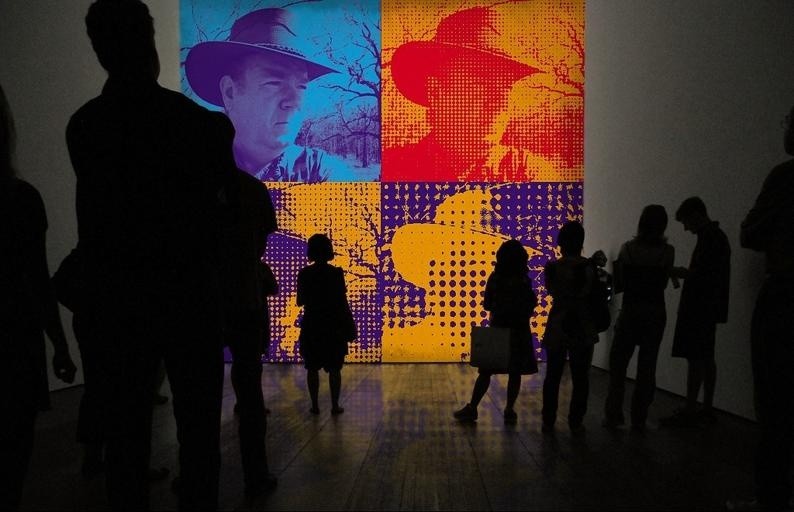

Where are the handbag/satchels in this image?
left=49, top=246, right=83, bottom=313
left=469, top=326, right=511, bottom=370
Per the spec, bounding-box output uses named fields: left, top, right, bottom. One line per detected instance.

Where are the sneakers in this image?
left=248, top=473, right=278, bottom=505
left=155, top=393, right=169, bottom=405
left=727, top=495, right=766, bottom=511
left=309, top=408, right=319, bottom=414
left=601, top=407, right=690, bottom=435
left=331, top=408, right=344, bottom=414
left=453, top=403, right=516, bottom=422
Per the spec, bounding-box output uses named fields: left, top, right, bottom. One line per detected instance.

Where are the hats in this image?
left=675, top=196, right=708, bottom=222
left=185, top=7, right=342, bottom=107
left=392, top=191, right=545, bottom=288
left=391, top=7, right=548, bottom=106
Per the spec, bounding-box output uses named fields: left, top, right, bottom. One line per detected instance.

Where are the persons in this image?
left=298, top=235, right=358, bottom=415
left=0, top=85, right=77, bottom=512
left=605, top=205, right=674, bottom=426
left=453, top=240, right=537, bottom=420
left=726, top=109, right=794, bottom=512
left=212, top=113, right=278, bottom=512
left=381, top=7, right=564, bottom=184
left=541, top=221, right=612, bottom=437
left=186, top=9, right=374, bottom=184
left=54, top=1, right=236, bottom=512
left=657, top=196, right=731, bottom=429
